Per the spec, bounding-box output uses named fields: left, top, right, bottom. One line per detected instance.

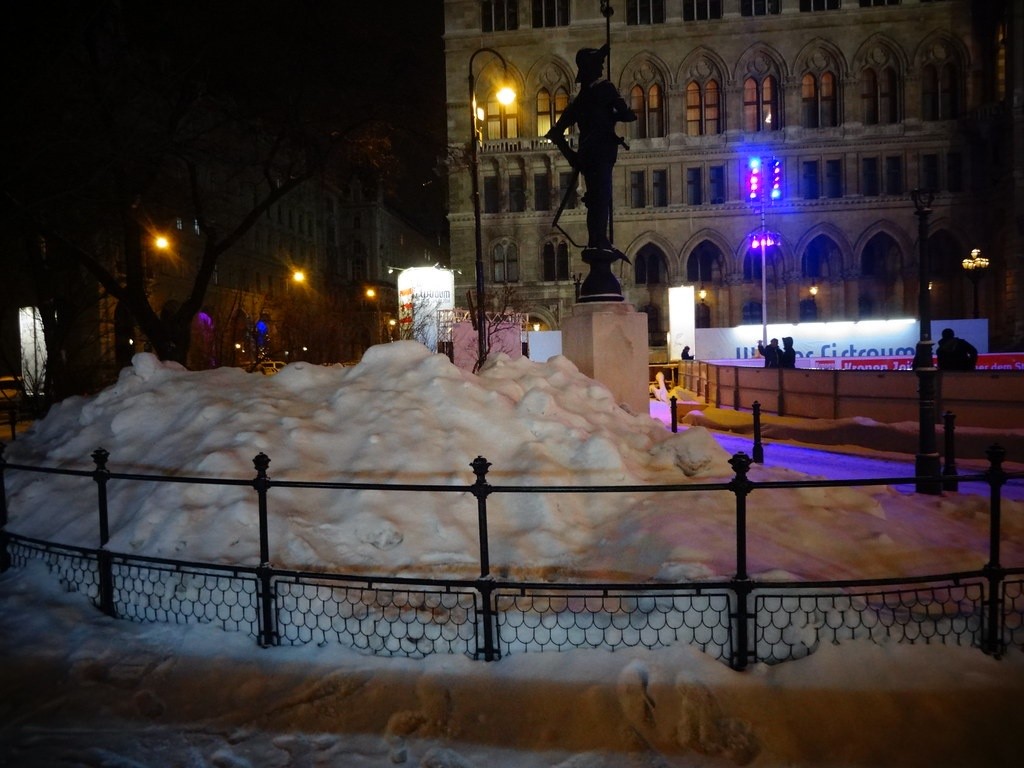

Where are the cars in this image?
left=260, top=361, right=287, bottom=376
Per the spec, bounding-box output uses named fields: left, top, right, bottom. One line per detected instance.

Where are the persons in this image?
left=545, top=41, right=639, bottom=251
left=682, top=347, right=696, bottom=360
left=934, top=327, right=980, bottom=373
left=757, top=336, right=796, bottom=370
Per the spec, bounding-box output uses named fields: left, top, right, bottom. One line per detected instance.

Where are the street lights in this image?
left=468, top=48, right=518, bottom=368
left=961, top=249, right=990, bottom=319
left=911, top=187, right=935, bottom=369
left=748, top=157, right=781, bottom=323
